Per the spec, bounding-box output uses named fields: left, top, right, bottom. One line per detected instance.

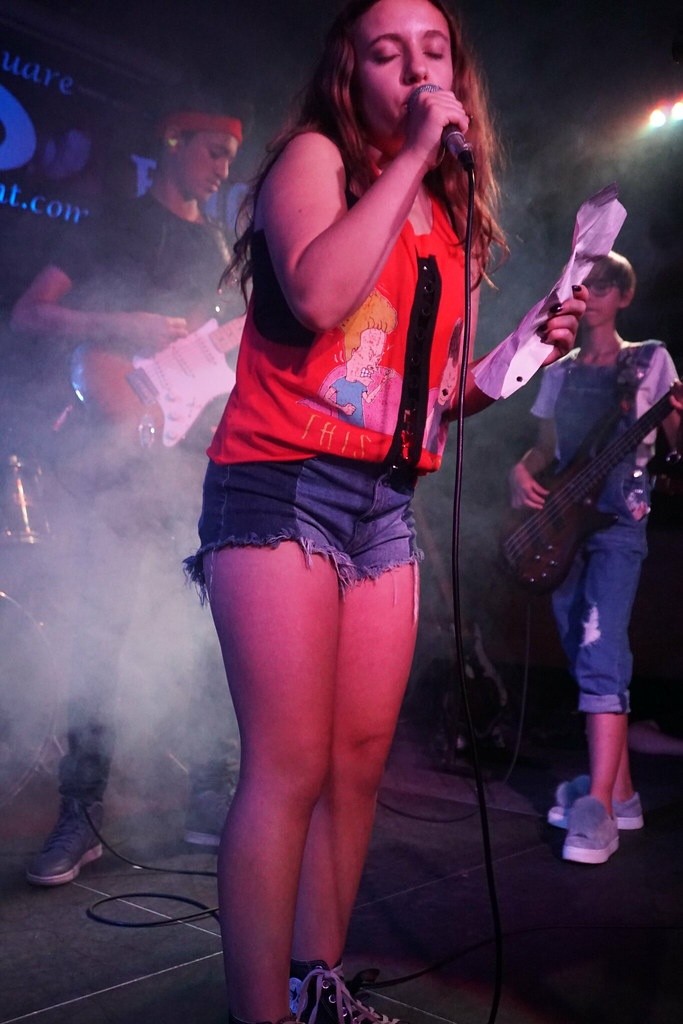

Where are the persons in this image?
left=508, top=248, right=683, bottom=864
left=13, top=93, right=247, bottom=888
left=183, top=0, right=508, bottom=1020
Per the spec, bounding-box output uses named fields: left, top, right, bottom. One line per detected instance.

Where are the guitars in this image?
left=67, top=306, right=247, bottom=468
left=498, top=378, right=681, bottom=587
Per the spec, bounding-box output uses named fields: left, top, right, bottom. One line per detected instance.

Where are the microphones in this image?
left=410, top=84, right=473, bottom=173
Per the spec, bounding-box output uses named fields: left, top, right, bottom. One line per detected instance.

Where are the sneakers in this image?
left=178, top=787, right=234, bottom=846
left=547, top=776, right=644, bottom=830
left=564, top=799, right=619, bottom=865
left=288, top=956, right=407, bottom=1024
left=23, top=795, right=107, bottom=885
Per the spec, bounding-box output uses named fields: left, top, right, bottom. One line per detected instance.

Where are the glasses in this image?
left=580, top=280, right=627, bottom=296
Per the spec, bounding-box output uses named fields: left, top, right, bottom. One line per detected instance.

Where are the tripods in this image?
left=344, top=177, right=608, bottom=1024
